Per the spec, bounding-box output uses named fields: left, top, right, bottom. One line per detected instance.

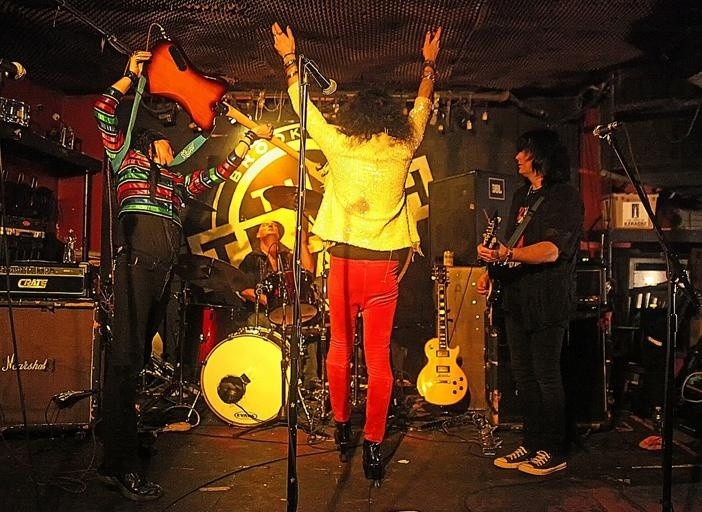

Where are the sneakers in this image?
left=518, top=448, right=568, bottom=476
left=493, top=445, right=534, bottom=470
left=94, top=469, right=164, bottom=501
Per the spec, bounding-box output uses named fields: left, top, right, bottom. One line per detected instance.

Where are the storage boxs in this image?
left=600, top=193, right=660, bottom=230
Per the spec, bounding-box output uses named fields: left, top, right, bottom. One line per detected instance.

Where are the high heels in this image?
left=362, top=439, right=385, bottom=489
left=333, top=419, right=352, bottom=463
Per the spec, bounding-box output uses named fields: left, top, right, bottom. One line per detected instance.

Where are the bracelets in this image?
left=239, top=140, right=250, bottom=150
left=244, top=130, right=259, bottom=143
left=504, top=247, right=513, bottom=264
left=419, top=59, right=438, bottom=82
left=124, top=71, right=139, bottom=84
left=280, top=53, right=298, bottom=79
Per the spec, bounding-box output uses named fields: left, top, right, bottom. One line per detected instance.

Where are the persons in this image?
left=477, top=127, right=586, bottom=476
left=96, top=52, right=271, bottom=499
left=236, top=220, right=316, bottom=327
left=271, top=21, right=440, bottom=481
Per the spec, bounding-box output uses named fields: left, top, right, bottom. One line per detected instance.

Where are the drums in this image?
left=200, top=327, right=301, bottom=427
left=183, top=303, right=237, bottom=364
left=266, top=271, right=318, bottom=325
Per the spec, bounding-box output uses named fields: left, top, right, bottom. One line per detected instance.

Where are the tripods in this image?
left=137, top=280, right=204, bottom=419
left=233, top=272, right=331, bottom=439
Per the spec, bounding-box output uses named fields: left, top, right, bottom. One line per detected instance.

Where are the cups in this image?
left=0, top=96, right=30, bottom=128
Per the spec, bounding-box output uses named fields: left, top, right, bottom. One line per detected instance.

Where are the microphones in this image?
left=593, top=121, right=622, bottom=136
left=274, top=240, right=282, bottom=271
left=300, top=54, right=337, bottom=96
left=52, top=390, right=96, bottom=401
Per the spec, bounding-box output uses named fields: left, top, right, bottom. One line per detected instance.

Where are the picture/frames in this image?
left=488, top=177, right=505, bottom=201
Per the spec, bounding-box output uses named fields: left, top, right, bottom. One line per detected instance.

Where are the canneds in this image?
left=442, top=250, right=454, bottom=267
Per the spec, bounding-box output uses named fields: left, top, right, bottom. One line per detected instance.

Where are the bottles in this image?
left=652, top=406, right=664, bottom=436
left=63, top=228, right=78, bottom=264
left=443, top=247, right=454, bottom=267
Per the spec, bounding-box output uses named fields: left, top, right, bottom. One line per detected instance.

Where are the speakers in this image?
left=0, top=298, right=108, bottom=432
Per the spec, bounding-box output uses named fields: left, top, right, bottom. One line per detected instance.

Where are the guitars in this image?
left=482, top=209, right=501, bottom=301
left=141, top=39, right=326, bottom=184
left=416, top=264, right=468, bottom=406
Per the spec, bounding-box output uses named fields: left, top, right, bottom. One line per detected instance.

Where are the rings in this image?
left=273, top=31, right=277, bottom=36
left=279, top=31, right=283, bottom=35
left=134, top=52, right=138, bottom=55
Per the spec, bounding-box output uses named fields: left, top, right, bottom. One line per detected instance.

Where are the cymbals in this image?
left=263, top=186, right=323, bottom=210
left=182, top=196, right=216, bottom=211
left=175, top=254, right=250, bottom=291
